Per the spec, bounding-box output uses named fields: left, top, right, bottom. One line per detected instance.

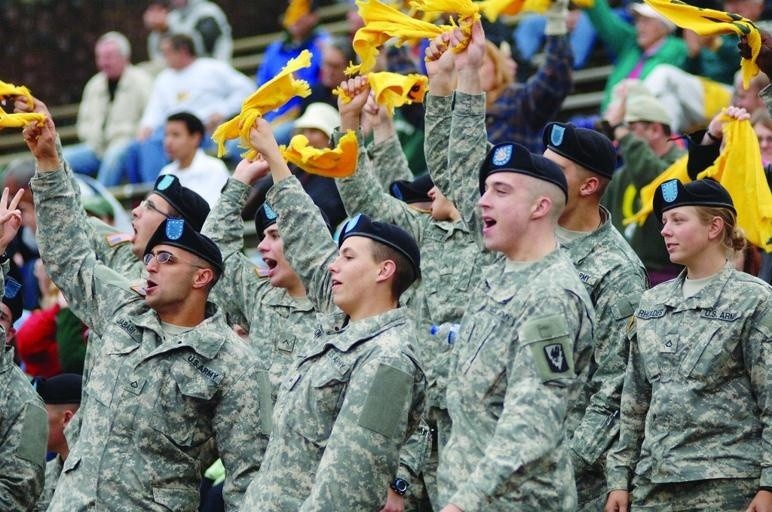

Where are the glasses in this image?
left=140, top=200, right=170, bottom=218
left=142, top=252, right=206, bottom=271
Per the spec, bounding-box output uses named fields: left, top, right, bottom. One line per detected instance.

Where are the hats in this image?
left=543, top=121, right=616, bottom=181
left=652, top=175, right=737, bottom=223
left=338, top=213, right=420, bottom=280
left=480, top=141, right=569, bottom=205
left=1, top=274, right=23, bottom=320
left=154, top=174, right=211, bottom=232
left=385, top=172, right=438, bottom=204
left=255, top=198, right=332, bottom=234
left=623, top=94, right=671, bottom=127
left=290, top=102, right=344, bottom=143
left=34, top=373, right=85, bottom=405
left=146, top=218, right=223, bottom=272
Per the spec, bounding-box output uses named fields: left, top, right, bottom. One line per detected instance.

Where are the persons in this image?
left=0, top=2, right=772, bottom=512
